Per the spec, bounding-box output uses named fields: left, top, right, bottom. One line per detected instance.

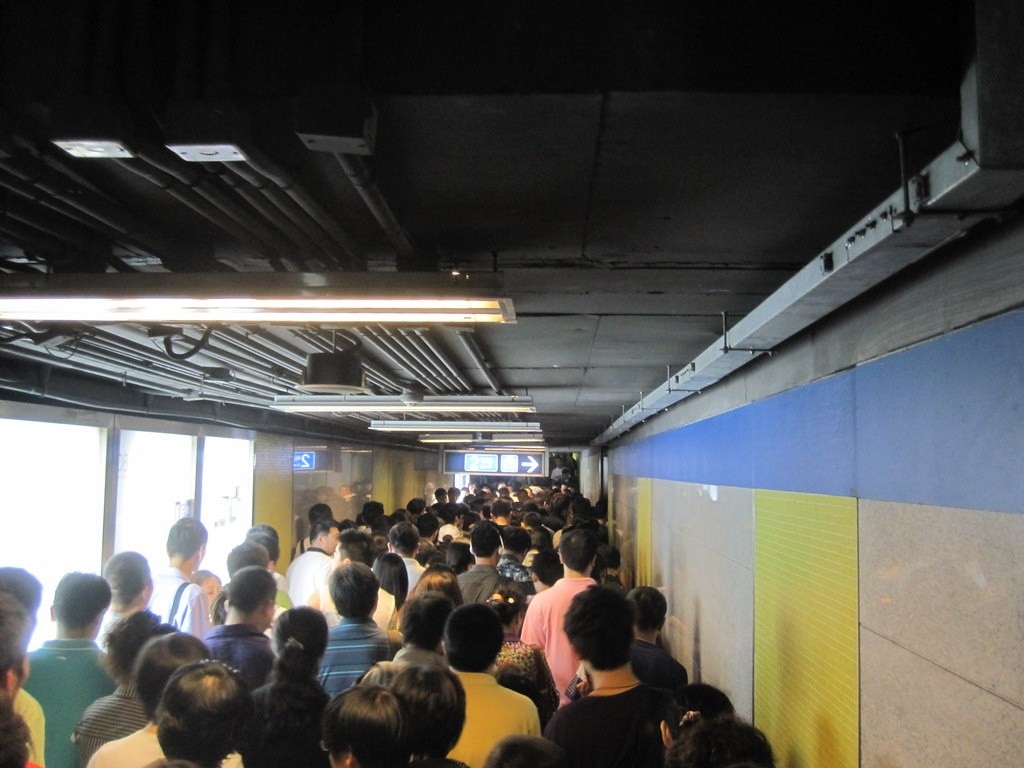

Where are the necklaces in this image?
left=592, top=681, right=641, bottom=691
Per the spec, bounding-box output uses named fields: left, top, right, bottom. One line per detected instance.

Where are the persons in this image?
left=0, top=451, right=780, bottom=768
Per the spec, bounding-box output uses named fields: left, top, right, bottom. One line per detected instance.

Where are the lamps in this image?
left=270, top=395, right=537, bottom=413
left=295, top=353, right=366, bottom=394
left=0, top=267, right=517, bottom=326
left=417, top=433, right=546, bottom=453
left=368, top=420, right=543, bottom=432
